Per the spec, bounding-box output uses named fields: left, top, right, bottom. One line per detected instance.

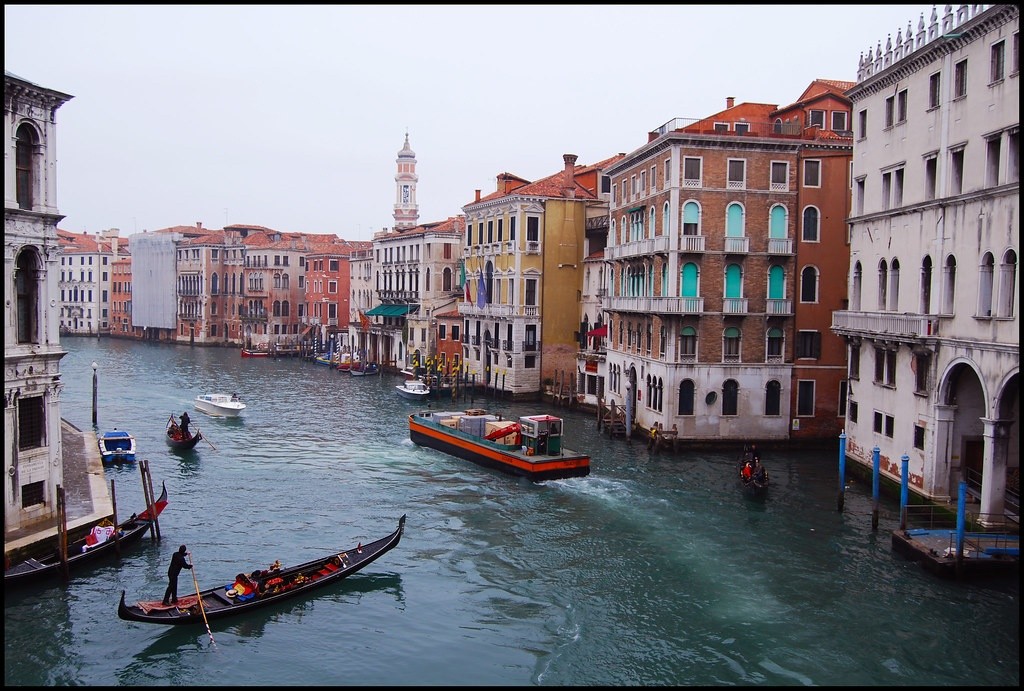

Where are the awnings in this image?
left=364, top=304, right=420, bottom=316
left=586, top=325, right=607, bottom=337
left=299, top=326, right=312, bottom=339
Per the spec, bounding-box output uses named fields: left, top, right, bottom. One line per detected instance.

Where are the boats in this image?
left=4, top=479, right=167, bottom=585
left=395, top=380, right=430, bottom=401
left=308, top=349, right=378, bottom=378
left=118, top=514, right=407, bottom=626
left=275, top=343, right=297, bottom=355
left=735, top=444, right=769, bottom=501
left=408, top=407, right=591, bottom=484
left=240, top=347, right=276, bottom=357
left=97, top=428, right=137, bottom=464
left=194, top=393, right=247, bottom=418
left=165, top=413, right=204, bottom=451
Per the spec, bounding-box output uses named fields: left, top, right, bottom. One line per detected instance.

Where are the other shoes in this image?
left=162, top=601, right=179, bottom=606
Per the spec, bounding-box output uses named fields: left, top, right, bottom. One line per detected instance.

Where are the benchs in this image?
left=100, top=436, right=134, bottom=440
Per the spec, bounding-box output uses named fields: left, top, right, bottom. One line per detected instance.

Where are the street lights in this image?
left=625, top=381, right=632, bottom=444
left=92, top=361, right=98, bottom=424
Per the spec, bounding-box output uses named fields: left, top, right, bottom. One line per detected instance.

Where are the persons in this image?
left=551, top=423, right=558, bottom=434
left=366, top=363, right=376, bottom=370
left=162, top=545, right=193, bottom=607
left=231, top=393, right=240, bottom=402
left=743, top=444, right=765, bottom=483
left=180, top=412, right=190, bottom=439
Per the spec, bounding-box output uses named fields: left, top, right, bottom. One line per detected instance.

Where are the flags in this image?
left=460, top=260, right=488, bottom=309
left=358, top=308, right=371, bottom=332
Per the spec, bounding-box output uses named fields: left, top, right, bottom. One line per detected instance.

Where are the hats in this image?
left=225, top=589, right=239, bottom=597
